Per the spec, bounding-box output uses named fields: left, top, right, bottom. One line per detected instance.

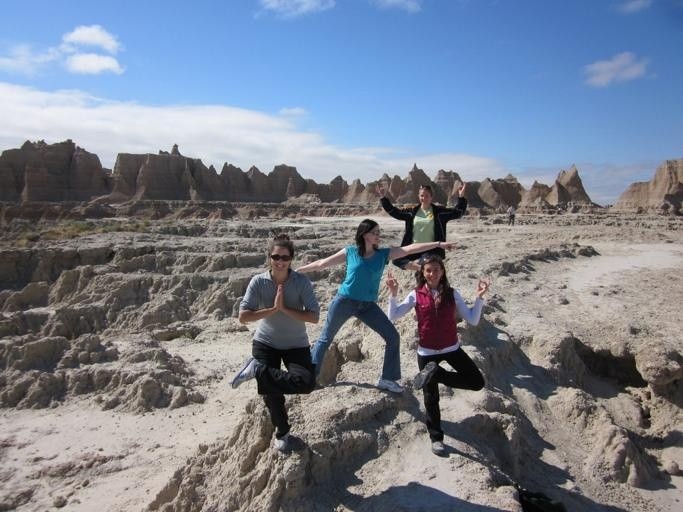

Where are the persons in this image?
left=231, top=230, right=320, bottom=452
left=293, top=218, right=458, bottom=394
left=375, top=182, right=467, bottom=286
left=385, top=255, right=491, bottom=455
left=507, top=205, right=515, bottom=226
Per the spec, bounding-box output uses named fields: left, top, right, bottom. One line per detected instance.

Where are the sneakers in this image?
left=272, top=425, right=291, bottom=451
left=432, top=439, right=445, bottom=456
left=230, top=356, right=258, bottom=391
left=374, top=376, right=406, bottom=393
left=413, top=359, right=437, bottom=391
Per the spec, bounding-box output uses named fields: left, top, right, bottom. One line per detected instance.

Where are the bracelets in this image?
left=437, top=241, right=441, bottom=245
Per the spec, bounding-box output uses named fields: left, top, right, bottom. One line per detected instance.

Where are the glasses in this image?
left=270, top=253, right=292, bottom=262
